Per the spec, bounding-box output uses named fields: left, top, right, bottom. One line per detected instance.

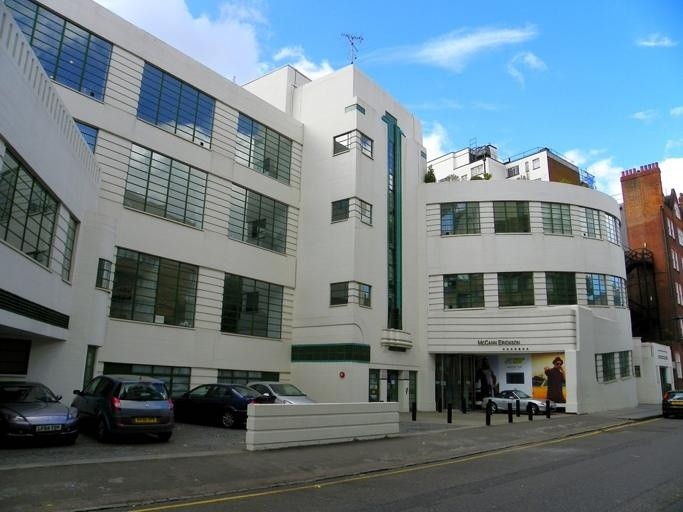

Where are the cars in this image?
left=173, top=383, right=277, bottom=428
left=246, top=381, right=318, bottom=404
left=662, top=391, right=683, bottom=417
left=0, top=381, right=80, bottom=447
left=482, top=390, right=557, bottom=414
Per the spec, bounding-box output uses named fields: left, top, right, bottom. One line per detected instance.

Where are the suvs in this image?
left=70, top=374, right=176, bottom=444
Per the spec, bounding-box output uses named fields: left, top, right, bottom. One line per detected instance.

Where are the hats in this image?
left=553, top=357, right=563, bottom=365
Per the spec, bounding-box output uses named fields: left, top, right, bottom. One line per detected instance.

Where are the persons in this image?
left=471, top=354, right=497, bottom=398
left=543, top=357, right=567, bottom=404
